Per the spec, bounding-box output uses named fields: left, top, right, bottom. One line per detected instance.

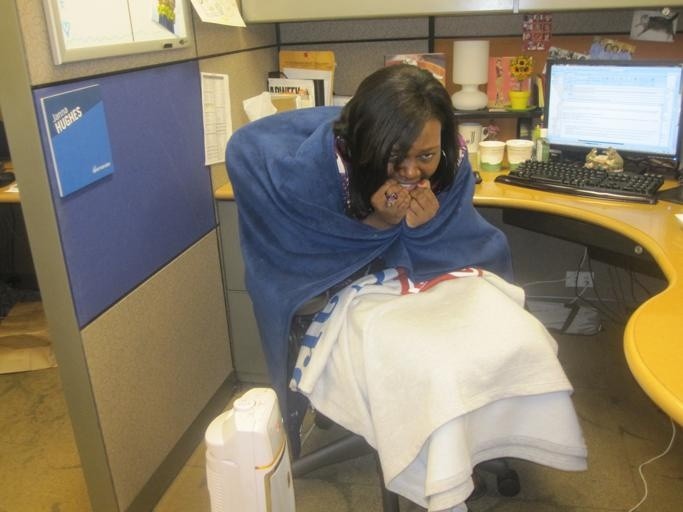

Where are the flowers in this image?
left=510, top=54, right=533, bottom=92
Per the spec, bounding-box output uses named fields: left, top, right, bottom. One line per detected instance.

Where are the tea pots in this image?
left=458, top=123, right=489, bottom=154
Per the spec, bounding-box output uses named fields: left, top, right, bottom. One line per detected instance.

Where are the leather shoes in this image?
left=467, top=464, right=520, bottom=501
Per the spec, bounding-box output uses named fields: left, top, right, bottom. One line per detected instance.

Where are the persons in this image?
left=285, top=63, right=590, bottom=510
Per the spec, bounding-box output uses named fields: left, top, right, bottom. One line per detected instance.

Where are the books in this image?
left=382, top=50, right=447, bottom=88
left=486, top=55, right=532, bottom=111
left=262, top=48, right=337, bottom=113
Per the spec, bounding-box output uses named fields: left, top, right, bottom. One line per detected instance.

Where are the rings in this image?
left=382, top=191, right=399, bottom=207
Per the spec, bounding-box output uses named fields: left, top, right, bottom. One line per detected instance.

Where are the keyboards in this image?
left=494, top=159, right=665, bottom=203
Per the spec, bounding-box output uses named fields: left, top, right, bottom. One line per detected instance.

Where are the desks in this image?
left=1, top=159, right=22, bottom=204
left=468, top=155, right=683, bottom=430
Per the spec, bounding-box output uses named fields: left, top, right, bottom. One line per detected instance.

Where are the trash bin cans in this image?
left=204, top=388, right=296, bottom=512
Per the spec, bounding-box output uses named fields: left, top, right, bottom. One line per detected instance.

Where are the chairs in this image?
left=288, top=292, right=520, bottom=511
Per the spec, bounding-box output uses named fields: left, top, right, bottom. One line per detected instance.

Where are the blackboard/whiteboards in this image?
left=41, top=0, right=195, bottom=65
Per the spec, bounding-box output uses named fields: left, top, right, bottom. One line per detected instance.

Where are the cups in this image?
left=480, top=139, right=535, bottom=171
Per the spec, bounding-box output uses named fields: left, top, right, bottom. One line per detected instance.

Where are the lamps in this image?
left=452, top=39, right=490, bottom=111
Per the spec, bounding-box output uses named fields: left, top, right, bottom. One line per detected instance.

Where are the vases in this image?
left=508, top=91, right=531, bottom=111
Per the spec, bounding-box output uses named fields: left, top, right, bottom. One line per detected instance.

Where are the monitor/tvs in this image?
left=543, top=58, right=683, bottom=170
